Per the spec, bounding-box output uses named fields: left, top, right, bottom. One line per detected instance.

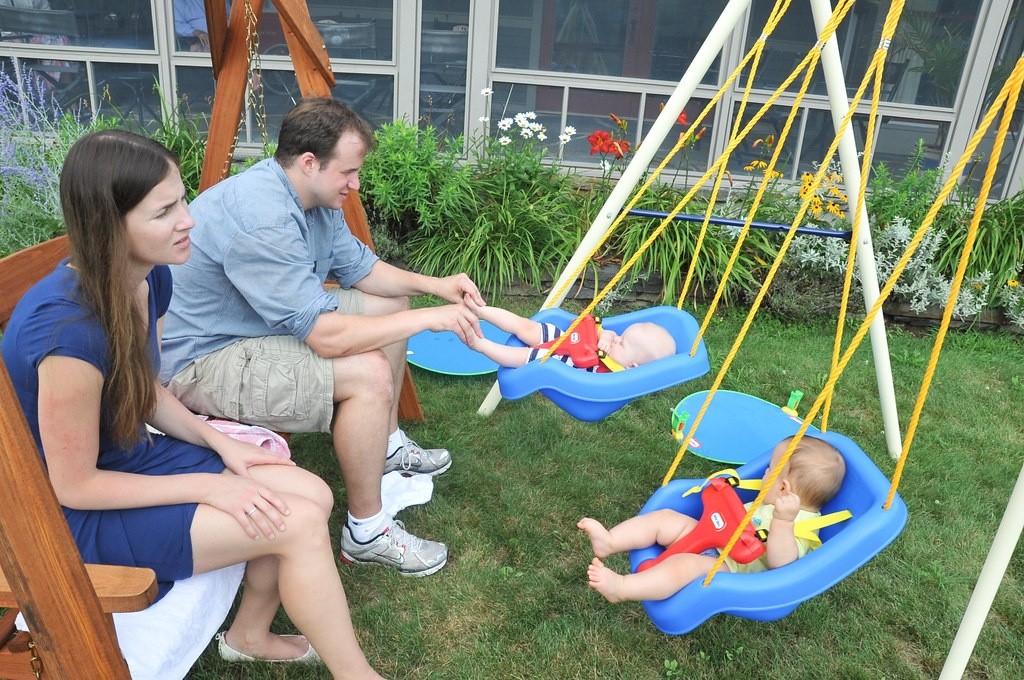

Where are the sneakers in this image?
left=339, top=520, right=448, bottom=577
left=382, top=428, right=452, bottom=477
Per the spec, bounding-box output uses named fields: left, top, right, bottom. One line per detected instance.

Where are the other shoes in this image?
left=215, top=629, right=322, bottom=664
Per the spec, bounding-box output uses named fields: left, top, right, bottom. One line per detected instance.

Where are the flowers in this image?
left=588, top=102, right=706, bottom=168
left=744, top=135, right=783, bottom=180
left=799, top=172, right=847, bottom=219
left=497, top=110, right=575, bottom=146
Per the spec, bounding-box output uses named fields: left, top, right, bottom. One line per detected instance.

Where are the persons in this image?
left=0, top=126, right=404, bottom=680
left=575, top=435, right=849, bottom=603
left=0, top=1, right=59, bottom=90
left=459, top=282, right=676, bottom=377
left=166, top=0, right=234, bottom=59
left=130, top=97, right=485, bottom=581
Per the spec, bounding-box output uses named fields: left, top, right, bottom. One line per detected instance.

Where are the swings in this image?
left=632, top=0, right=1024, bottom=636
left=499, top=0, right=855, bottom=421
